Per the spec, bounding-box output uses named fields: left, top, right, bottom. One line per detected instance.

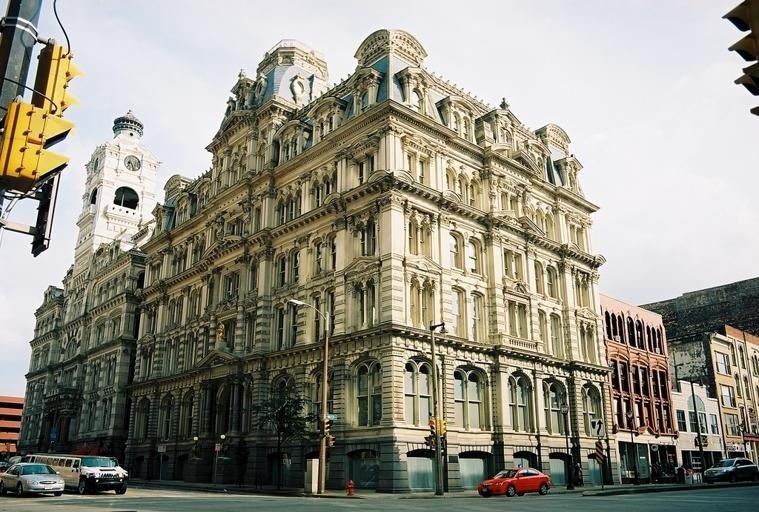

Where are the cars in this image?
left=0, top=462, right=65, bottom=497
left=477, top=467, right=553, bottom=497
left=704, top=457, right=759, bottom=483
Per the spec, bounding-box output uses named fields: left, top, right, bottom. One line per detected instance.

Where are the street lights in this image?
left=689, top=376, right=707, bottom=483
left=219, top=433, right=227, bottom=457
left=289, top=298, right=330, bottom=493
left=192, top=435, right=200, bottom=459
left=560, top=400, right=575, bottom=490
left=627, top=409, right=640, bottom=484
left=429, top=322, right=450, bottom=495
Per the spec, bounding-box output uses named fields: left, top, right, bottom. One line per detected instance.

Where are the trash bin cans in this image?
left=677, top=467, right=692, bottom=484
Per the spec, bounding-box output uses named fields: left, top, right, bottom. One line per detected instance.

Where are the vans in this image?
left=24, top=450, right=129, bottom=495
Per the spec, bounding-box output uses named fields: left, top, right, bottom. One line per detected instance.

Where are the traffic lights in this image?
left=440, top=418, right=446, bottom=436
left=429, top=419, right=436, bottom=435
left=31, top=44, right=85, bottom=118
left=0, top=99, right=74, bottom=194
left=721, top=1, right=759, bottom=98
left=424, top=436, right=436, bottom=447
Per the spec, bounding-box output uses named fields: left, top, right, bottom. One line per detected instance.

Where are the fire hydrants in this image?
left=346, top=478, right=355, bottom=497
